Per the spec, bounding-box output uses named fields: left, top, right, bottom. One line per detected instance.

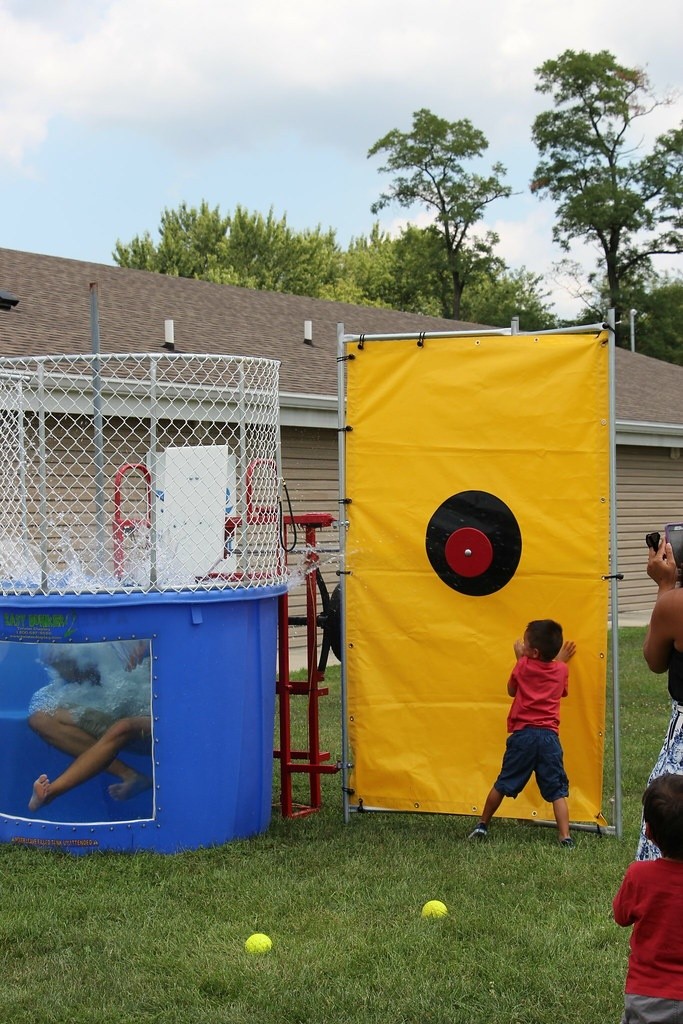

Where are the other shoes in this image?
left=561, top=838, right=573, bottom=848
left=468, top=821, right=487, bottom=838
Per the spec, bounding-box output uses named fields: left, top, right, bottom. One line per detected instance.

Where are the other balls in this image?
left=245, top=932, right=273, bottom=957
left=422, top=900, right=446, bottom=921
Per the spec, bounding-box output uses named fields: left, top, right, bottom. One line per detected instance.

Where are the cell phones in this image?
left=665, top=522, right=683, bottom=587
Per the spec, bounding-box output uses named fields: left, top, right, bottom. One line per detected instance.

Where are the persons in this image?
left=612, top=773, right=683, bottom=1024
left=634, top=536, right=683, bottom=860
left=26, top=641, right=152, bottom=812
left=468, top=619, right=577, bottom=850
